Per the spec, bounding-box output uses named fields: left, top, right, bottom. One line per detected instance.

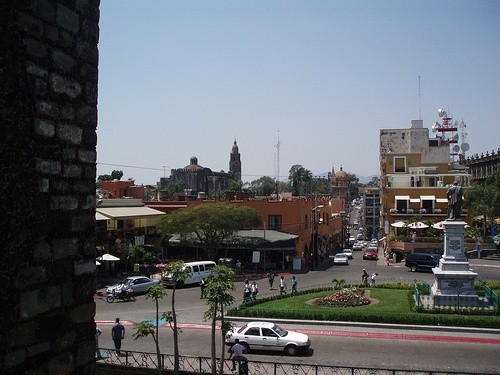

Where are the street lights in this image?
left=311, top=204, right=324, bottom=272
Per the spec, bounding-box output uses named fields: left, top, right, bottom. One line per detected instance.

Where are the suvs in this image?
left=405, top=253, right=443, bottom=272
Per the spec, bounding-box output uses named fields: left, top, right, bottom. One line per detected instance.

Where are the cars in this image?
left=349, top=228, right=377, bottom=251
left=225, top=322, right=311, bottom=357
left=333, top=253, right=349, bottom=265
left=363, top=248, right=377, bottom=260
left=343, top=248, right=353, bottom=259
left=106, top=276, right=160, bottom=296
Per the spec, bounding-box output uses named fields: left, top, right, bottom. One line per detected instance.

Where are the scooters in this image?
left=106, top=289, right=131, bottom=303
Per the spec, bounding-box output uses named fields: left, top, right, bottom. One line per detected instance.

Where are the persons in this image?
left=242, top=279, right=258, bottom=303
left=113, top=281, right=125, bottom=300
left=227, top=339, right=250, bottom=371
left=236, top=259, right=242, bottom=275
left=267, top=270, right=274, bottom=290
left=95, top=328, right=102, bottom=358
left=325, top=245, right=330, bottom=257
left=319, top=251, right=325, bottom=263
left=285, top=254, right=290, bottom=270
left=446, top=181, right=464, bottom=219
left=111, top=318, right=125, bottom=356
left=291, top=276, right=297, bottom=292
left=386, top=254, right=389, bottom=261
left=198, top=278, right=208, bottom=299
left=279, top=276, right=287, bottom=295
left=361, top=269, right=378, bottom=284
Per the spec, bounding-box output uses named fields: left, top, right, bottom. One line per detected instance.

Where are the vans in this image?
left=161, top=261, right=218, bottom=289
